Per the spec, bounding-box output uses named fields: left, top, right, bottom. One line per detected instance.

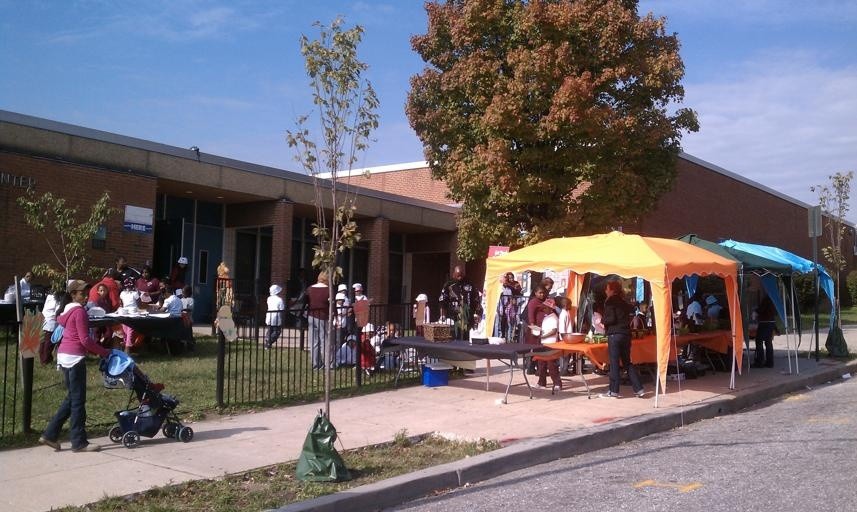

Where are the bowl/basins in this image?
left=115, top=306, right=137, bottom=315
left=560, top=332, right=586, bottom=344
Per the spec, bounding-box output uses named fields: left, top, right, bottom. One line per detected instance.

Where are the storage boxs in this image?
left=421, top=364, right=453, bottom=386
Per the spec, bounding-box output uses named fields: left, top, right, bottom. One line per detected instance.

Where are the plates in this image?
left=4, top=293, right=16, bottom=302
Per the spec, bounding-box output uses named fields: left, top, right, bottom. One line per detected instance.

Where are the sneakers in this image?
left=605, top=390, right=620, bottom=397
left=72, top=442, right=100, bottom=453
left=633, top=388, right=644, bottom=397
left=750, top=361, right=772, bottom=368
left=38, top=436, right=61, bottom=450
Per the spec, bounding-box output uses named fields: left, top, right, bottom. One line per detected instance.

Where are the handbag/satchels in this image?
left=51, top=323, right=64, bottom=344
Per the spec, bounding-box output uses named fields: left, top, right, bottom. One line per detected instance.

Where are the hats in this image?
left=269, top=285, right=282, bottom=295
left=335, top=283, right=363, bottom=300
left=347, top=335, right=356, bottom=341
left=706, top=295, right=717, bottom=305
left=415, top=292, right=429, bottom=303
left=68, top=280, right=91, bottom=291
left=178, top=256, right=188, bottom=264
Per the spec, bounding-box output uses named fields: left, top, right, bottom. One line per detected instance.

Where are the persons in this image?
left=439, top=259, right=483, bottom=373
left=413, top=294, right=430, bottom=337
left=492, top=272, right=732, bottom=397
left=360, top=322, right=410, bottom=376
left=686, top=287, right=780, bottom=372
left=37, top=279, right=113, bottom=452
left=40, top=290, right=65, bottom=365
left=265, top=285, right=288, bottom=350
left=20, top=271, right=34, bottom=297
left=85, top=256, right=195, bottom=360
left=284, top=267, right=370, bottom=369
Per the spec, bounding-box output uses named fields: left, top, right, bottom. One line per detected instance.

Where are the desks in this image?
left=381, top=337, right=543, bottom=404
left=544, top=324, right=774, bottom=399
left=90, top=313, right=181, bottom=359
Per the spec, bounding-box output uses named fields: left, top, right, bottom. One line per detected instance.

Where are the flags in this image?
left=474, top=245, right=509, bottom=335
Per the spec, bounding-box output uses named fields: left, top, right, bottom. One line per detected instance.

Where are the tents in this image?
left=674, top=233, right=800, bottom=376
left=483, top=230, right=744, bottom=408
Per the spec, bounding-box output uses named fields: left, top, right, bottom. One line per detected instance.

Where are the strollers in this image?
left=97, top=347, right=195, bottom=451
